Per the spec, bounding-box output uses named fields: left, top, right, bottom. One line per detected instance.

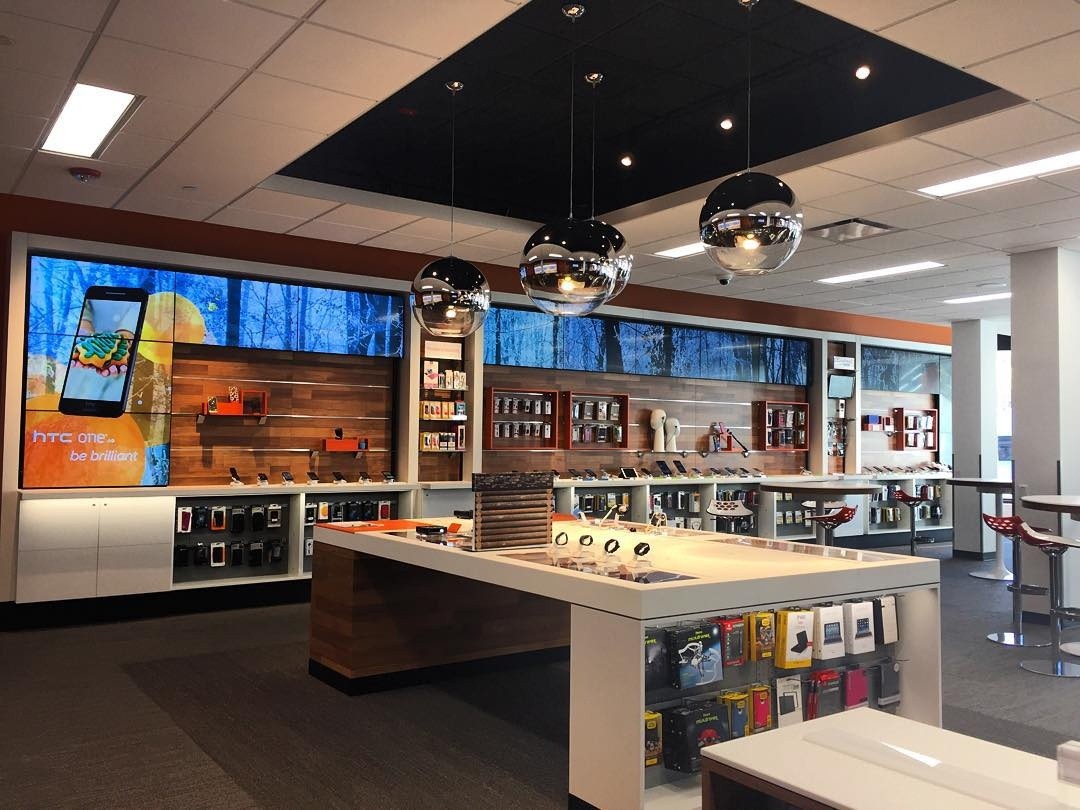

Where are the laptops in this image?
left=824, top=622, right=843, bottom=644
left=854, top=618, right=873, bottom=638
left=791, top=631, right=808, bottom=653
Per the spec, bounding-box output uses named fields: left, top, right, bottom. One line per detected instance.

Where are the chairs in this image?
left=892, top=490, right=932, bottom=556
left=983, top=513, right=1053, bottom=647
left=1017, top=522, right=1080, bottom=678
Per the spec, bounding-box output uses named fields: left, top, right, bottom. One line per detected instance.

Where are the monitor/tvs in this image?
left=827, top=369, right=856, bottom=399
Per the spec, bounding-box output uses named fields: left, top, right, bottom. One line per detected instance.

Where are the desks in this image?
left=946, top=478, right=1013, bottom=581
left=698, top=707, right=1080, bottom=810
left=308, top=516, right=943, bottom=810
left=760, top=482, right=882, bottom=545
left=1021, top=495, right=1080, bottom=656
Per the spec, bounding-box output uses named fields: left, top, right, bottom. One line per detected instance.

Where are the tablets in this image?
left=673, top=460, right=687, bottom=473
left=620, top=467, right=639, bottom=478
left=655, top=460, right=672, bottom=474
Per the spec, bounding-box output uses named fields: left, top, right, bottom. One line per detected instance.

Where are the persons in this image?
left=650, top=408, right=681, bottom=450
left=63, top=299, right=143, bottom=402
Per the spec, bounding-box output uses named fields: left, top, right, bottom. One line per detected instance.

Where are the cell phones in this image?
left=208, top=396, right=218, bottom=413
left=58, top=285, right=149, bottom=418
left=335, top=427, right=343, bottom=439
left=641, top=468, right=652, bottom=475
left=230, top=467, right=240, bottom=480
left=333, top=472, right=345, bottom=481
left=258, top=473, right=268, bottom=481
left=359, top=472, right=370, bottom=479
left=531, top=469, right=597, bottom=477
left=692, top=468, right=762, bottom=473
left=282, top=472, right=293, bottom=480
left=306, top=472, right=319, bottom=480
left=381, top=471, right=395, bottom=479
left=251, top=397, right=260, bottom=413
left=863, top=466, right=935, bottom=472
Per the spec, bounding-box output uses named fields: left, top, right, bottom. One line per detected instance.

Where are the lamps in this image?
left=410, top=82, right=491, bottom=337
left=519, top=5, right=616, bottom=317
left=582, top=73, right=634, bottom=304
left=697, top=0, right=804, bottom=277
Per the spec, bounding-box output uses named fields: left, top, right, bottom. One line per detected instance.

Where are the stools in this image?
left=801, top=500, right=858, bottom=546
left=706, top=499, right=754, bottom=535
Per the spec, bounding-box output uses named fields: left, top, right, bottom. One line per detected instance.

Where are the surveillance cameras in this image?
left=716, top=274, right=732, bottom=286
